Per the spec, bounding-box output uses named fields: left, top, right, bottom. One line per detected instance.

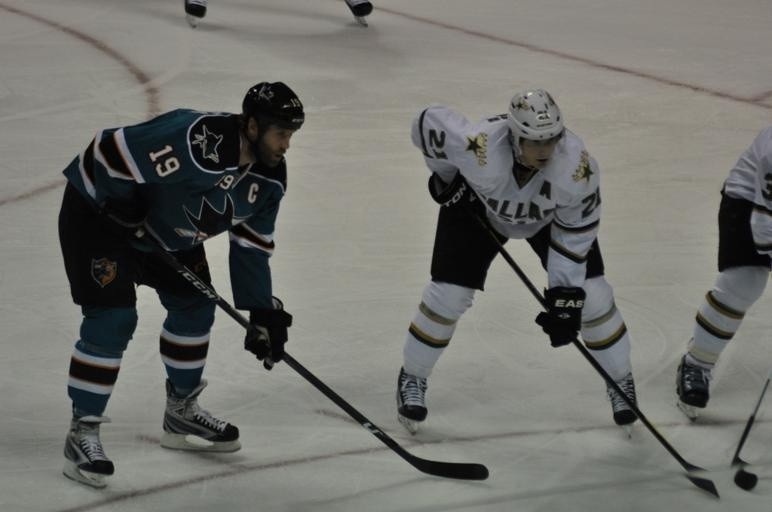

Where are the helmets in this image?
left=241, top=80, right=306, bottom=133
left=505, top=89, right=564, bottom=142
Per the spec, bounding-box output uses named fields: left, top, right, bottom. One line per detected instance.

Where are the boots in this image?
left=161, top=377, right=239, bottom=442
left=64, top=401, right=116, bottom=475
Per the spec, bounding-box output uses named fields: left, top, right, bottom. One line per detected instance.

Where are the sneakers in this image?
left=395, top=365, right=431, bottom=422
left=345, top=1, right=374, bottom=18
left=184, top=0, right=206, bottom=18
left=675, top=353, right=711, bottom=408
left=606, top=373, right=640, bottom=425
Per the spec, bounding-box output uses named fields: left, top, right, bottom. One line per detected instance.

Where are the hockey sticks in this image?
left=732, top=377, right=772, bottom=492
left=157, top=244, right=490, bottom=478
left=478, top=213, right=721, bottom=501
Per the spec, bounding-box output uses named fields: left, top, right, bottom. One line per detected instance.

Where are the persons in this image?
left=395, top=88, right=639, bottom=425
left=58, top=80, right=306, bottom=474
left=186, top=0, right=375, bottom=18
left=676, top=124, right=772, bottom=407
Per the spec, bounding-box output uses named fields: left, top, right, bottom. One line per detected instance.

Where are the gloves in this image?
left=243, top=295, right=294, bottom=374
left=100, top=191, right=151, bottom=243
left=535, top=286, right=587, bottom=349
left=429, top=170, right=483, bottom=231
